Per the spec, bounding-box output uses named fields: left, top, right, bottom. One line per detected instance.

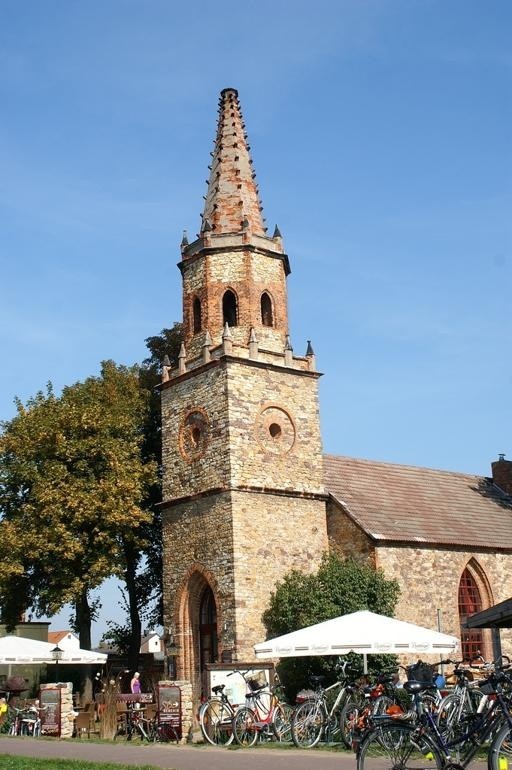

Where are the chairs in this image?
left=15, top=700, right=26, bottom=709
left=74, top=701, right=128, bottom=739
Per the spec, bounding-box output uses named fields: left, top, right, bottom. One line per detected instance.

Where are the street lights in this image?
left=51, top=644, right=63, bottom=682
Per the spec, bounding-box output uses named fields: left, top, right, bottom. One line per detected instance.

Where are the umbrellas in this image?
left=0, top=634, right=109, bottom=679
left=254, top=608, right=462, bottom=677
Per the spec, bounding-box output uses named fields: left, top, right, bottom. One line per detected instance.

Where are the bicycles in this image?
left=113, top=703, right=178, bottom=745
left=6, top=703, right=50, bottom=736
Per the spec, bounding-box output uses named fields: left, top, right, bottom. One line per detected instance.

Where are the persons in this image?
left=0, top=675, right=10, bottom=700
left=130, top=671, right=142, bottom=709
